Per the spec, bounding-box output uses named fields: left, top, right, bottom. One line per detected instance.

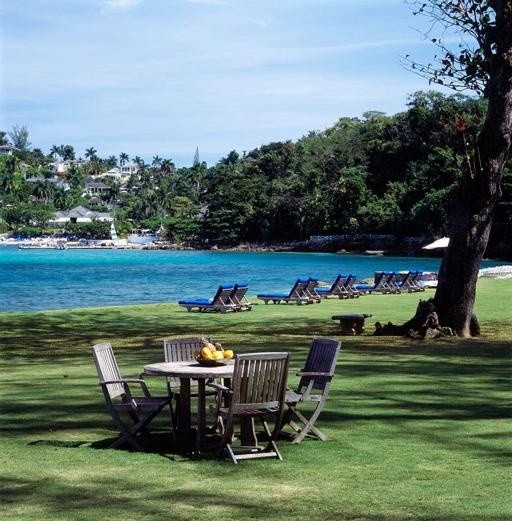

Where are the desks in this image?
left=144, top=361, right=271, bottom=459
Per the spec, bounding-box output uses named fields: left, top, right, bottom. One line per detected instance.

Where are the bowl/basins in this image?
left=193, top=352, right=233, bottom=366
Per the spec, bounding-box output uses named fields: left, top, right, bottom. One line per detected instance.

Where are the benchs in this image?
left=331, top=313, right=372, bottom=336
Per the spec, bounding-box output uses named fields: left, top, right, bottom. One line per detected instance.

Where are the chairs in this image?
left=271, top=337, right=342, bottom=444
left=162, top=336, right=226, bottom=434
left=178, top=268, right=427, bottom=310
left=16, top=236, right=92, bottom=250
left=91, top=342, right=173, bottom=453
left=208, top=350, right=291, bottom=465
